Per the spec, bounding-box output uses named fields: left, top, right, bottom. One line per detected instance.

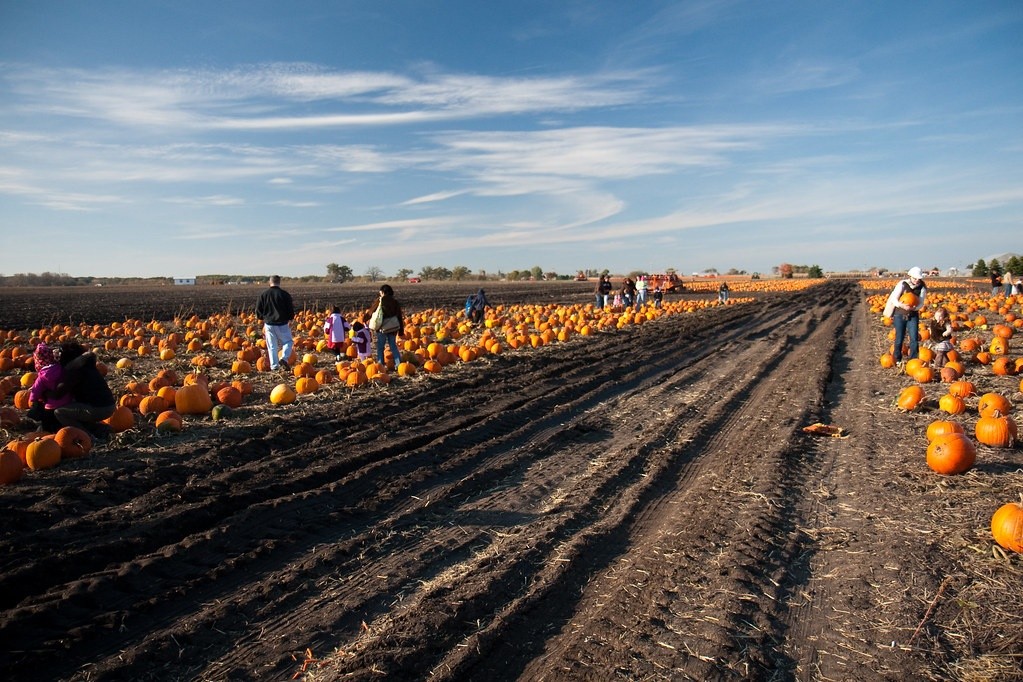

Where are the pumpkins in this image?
left=856, top=278, right=1023, bottom=474
left=0, top=298, right=755, bottom=488
left=681, top=279, right=829, bottom=292
left=991, top=492, right=1023, bottom=556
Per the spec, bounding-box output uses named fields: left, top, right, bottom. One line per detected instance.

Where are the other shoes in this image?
left=279, top=359, right=289, bottom=371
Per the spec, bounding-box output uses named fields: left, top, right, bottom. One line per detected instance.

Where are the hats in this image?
left=908, top=267, right=923, bottom=279
left=33, top=342, right=55, bottom=371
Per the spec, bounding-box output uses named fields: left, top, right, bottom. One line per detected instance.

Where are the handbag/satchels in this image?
left=369, top=297, right=383, bottom=331
left=1011, top=284, right=1018, bottom=295
left=380, top=315, right=400, bottom=333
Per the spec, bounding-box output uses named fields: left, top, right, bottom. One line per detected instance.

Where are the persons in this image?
left=375, top=284, right=404, bottom=371
left=351, top=322, right=372, bottom=361
left=255, top=276, right=295, bottom=370
left=891, top=267, right=925, bottom=365
left=27, top=341, right=114, bottom=439
left=653, top=286, right=663, bottom=309
left=465, top=294, right=476, bottom=319
left=1003, top=268, right=1013, bottom=299
left=717, top=283, right=729, bottom=301
left=1016, top=277, right=1023, bottom=293
left=990, top=268, right=1002, bottom=296
left=923, top=306, right=953, bottom=366
left=472, top=288, right=492, bottom=324
left=324, top=307, right=351, bottom=361
left=595, top=275, right=648, bottom=311
